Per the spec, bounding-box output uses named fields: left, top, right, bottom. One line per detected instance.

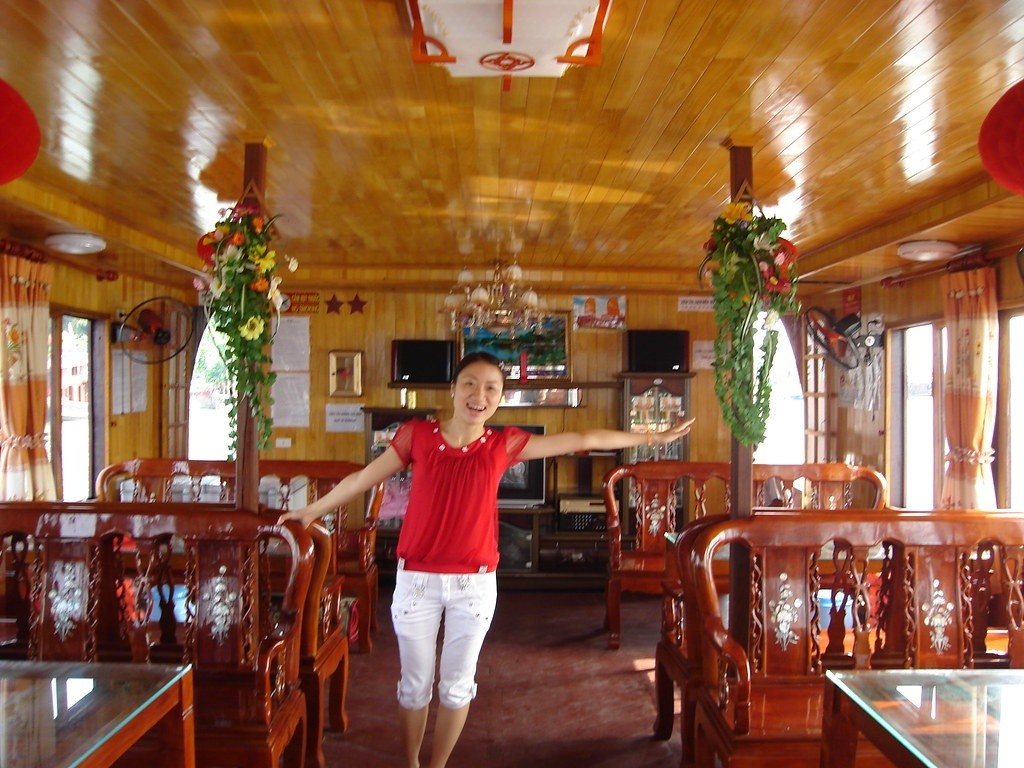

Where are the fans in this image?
left=112, top=297, right=194, bottom=364
left=804, top=306, right=862, bottom=370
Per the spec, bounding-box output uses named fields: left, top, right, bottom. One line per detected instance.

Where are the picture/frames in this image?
left=455, top=309, right=575, bottom=385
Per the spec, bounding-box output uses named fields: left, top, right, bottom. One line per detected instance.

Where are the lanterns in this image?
left=0, top=79, right=40, bottom=186
left=196, top=230, right=215, bottom=268
left=978, top=77, right=1024, bottom=201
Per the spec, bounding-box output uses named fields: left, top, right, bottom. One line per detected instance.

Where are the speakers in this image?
left=390, top=340, right=456, bottom=384
left=622, top=330, right=691, bottom=373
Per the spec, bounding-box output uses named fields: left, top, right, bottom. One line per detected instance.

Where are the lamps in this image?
left=438, top=261, right=545, bottom=339
left=898, top=240, right=958, bottom=261
left=45, top=233, right=106, bottom=254
left=405, top=0, right=611, bottom=77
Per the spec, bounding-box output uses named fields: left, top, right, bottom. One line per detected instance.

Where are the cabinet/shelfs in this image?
left=488, top=507, right=608, bottom=592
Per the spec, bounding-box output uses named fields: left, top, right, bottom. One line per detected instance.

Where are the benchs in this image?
left=0, top=460, right=385, bottom=768
left=602, top=461, right=1024, bottom=768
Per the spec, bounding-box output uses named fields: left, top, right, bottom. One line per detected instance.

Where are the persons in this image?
left=277, top=349, right=696, bottom=768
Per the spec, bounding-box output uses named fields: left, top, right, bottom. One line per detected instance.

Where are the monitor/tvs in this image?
left=490, top=424, right=547, bottom=509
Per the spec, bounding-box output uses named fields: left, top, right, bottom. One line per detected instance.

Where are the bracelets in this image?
left=647, top=434, right=654, bottom=448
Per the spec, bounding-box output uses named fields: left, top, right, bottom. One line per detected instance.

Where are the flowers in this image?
left=708, top=204, right=797, bottom=312
left=195, top=204, right=297, bottom=340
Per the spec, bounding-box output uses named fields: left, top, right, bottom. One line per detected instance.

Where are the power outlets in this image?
left=867, top=313, right=883, bottom=335
left=115, top=309, right=125, bottom=322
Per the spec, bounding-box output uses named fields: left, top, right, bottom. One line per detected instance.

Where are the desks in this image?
left=823, top=669, right=1024, bottom=768
left=0, top=658, right=197, bottom=768
